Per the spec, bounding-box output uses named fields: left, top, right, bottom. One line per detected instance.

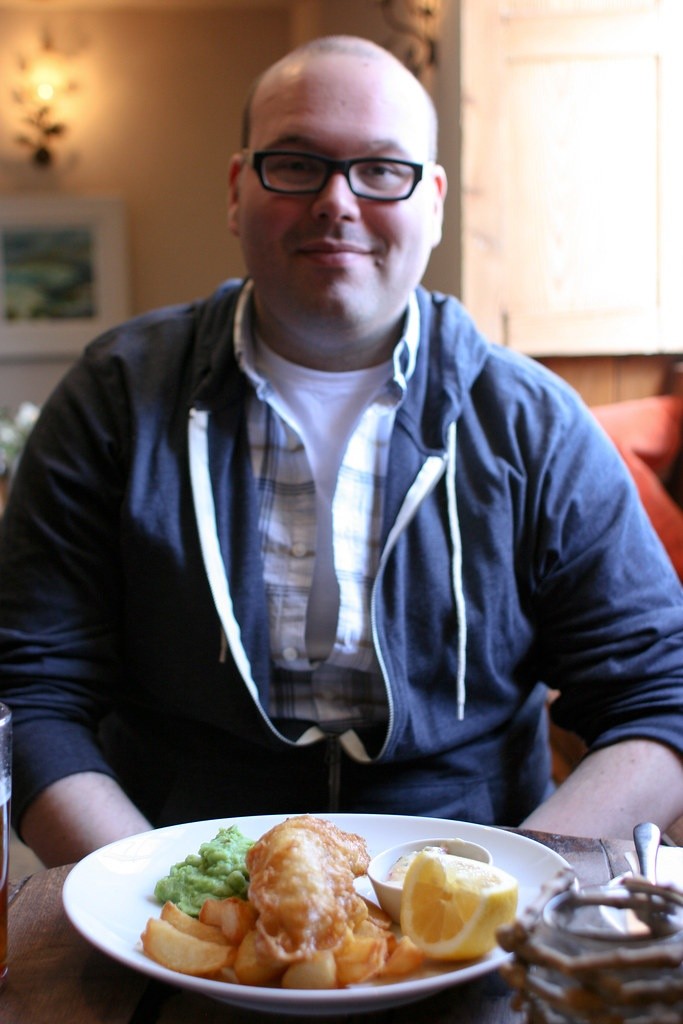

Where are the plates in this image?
left=62, top=813, right=580, bottom=1013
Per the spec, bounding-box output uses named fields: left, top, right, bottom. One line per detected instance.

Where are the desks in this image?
left=0, top=824, right=683, bottom=1024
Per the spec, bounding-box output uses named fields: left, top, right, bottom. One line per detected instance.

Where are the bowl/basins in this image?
left=366, top=838, right=492, bottom=923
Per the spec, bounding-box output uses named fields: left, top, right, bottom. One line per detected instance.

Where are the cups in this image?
left=0, top=701, right=11, bottom=981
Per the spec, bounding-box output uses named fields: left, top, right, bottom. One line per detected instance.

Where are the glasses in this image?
left=244, top=151, right=422, bottom=201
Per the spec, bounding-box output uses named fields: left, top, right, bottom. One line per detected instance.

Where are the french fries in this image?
left=140, top=896, right=422, bottom=991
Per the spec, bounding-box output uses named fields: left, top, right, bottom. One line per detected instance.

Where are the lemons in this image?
left=400, top=850, right=520, bottom=958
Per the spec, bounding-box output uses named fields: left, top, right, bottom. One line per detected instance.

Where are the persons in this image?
left=0, top=37, right=683, bottom=864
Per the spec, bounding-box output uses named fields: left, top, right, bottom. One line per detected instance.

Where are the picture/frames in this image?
left=0, top=197, right=133, bottom=360
